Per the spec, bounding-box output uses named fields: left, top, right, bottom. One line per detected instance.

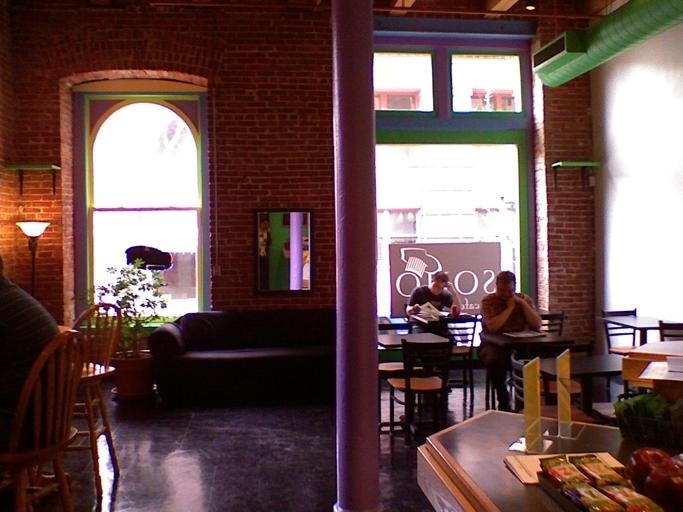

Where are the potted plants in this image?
left=71, top=258, right=171, bottom=401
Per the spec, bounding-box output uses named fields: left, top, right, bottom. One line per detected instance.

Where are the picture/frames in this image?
left=254, top=207, right=315, bottom=297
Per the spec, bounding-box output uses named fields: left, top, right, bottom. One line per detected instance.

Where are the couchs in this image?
left=149, top=307, right=336, bottom=404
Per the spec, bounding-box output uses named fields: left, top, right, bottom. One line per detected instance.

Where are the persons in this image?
left=0, top=256, right=61, bottom=451
left=406, top=272, right=461, bottom=376
left=478, top=271, right=542, bottom=411
left=257, top=218, right=309, bottom=290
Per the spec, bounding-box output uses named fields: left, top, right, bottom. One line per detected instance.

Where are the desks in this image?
left=416, top=410, right=683, bottom=512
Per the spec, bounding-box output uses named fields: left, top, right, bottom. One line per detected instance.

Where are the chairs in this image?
left=0, top=331, right=86, bottom=511
left=59, top=302, right=121, bottom=499
left=380, top=302, right=480, bottom=466
left=508, top=307, right=682, bottom=424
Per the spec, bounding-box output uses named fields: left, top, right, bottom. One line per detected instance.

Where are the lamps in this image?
left=15, top=221, right=52, bottom=298
